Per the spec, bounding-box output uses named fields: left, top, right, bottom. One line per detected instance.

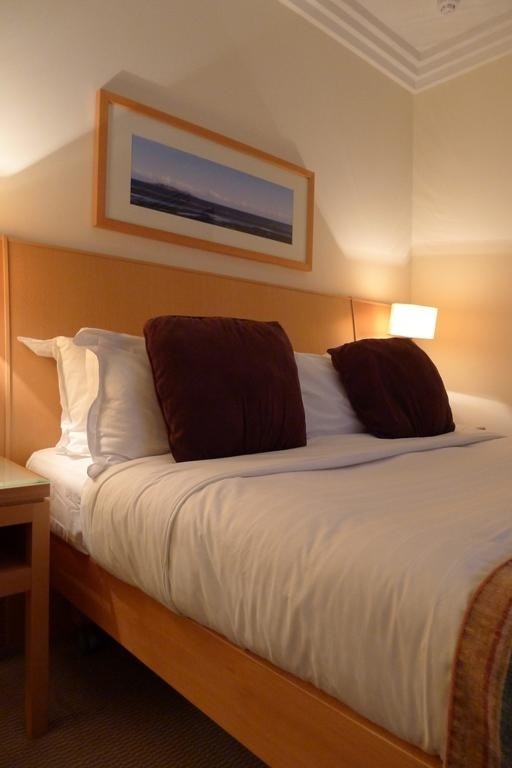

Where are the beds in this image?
left=4, top=234, right=508, bottom=768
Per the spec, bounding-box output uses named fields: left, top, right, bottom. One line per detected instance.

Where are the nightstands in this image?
left=2, top=453, right=58, bottom=738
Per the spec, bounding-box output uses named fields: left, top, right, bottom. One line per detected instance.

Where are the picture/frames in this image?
left=91, top=85, right=320, bottom=276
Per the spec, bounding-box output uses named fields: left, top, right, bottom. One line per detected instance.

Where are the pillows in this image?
left=18, top=315, right=457, bottom=480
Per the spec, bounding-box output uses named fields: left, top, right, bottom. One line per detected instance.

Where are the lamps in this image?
left=383, top=301, right=442, bottom=346
